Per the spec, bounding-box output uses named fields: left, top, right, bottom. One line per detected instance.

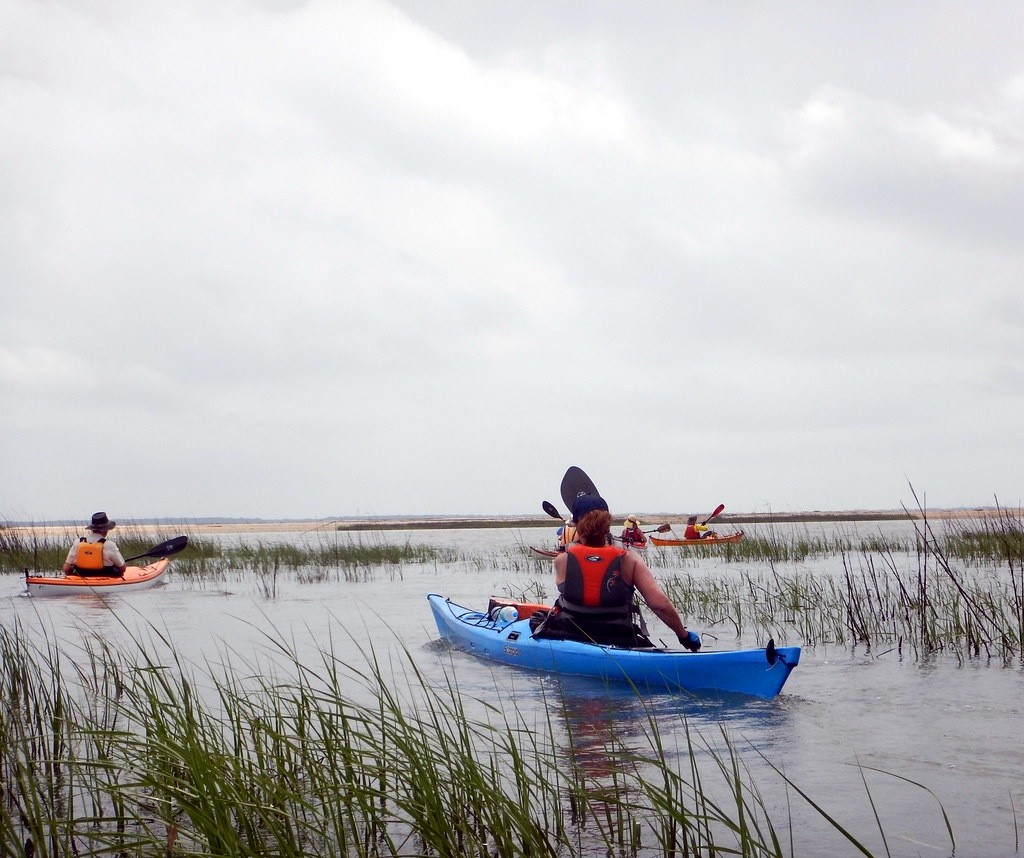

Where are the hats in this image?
left=624, top=515, right=640, bottom=529
left=85, top=511, right=116, bottom=532
left=573, top=494, right=608, bottom=522
left=688, top=516, right=697, bottom=524
left=565, top=513, right=576, bottom=527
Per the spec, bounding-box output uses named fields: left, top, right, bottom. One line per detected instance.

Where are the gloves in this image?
left=678, top=631, right=701, bottom=653
left=702, top=521, right=706, bottom=526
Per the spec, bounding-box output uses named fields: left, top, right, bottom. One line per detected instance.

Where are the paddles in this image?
left=559, top=465, right=649, bottom=606
left=542, top=501, right=565, bottom=522
left=700, top=503, right=725, bottom=526
left=642, top=523, right=671, bottom=534
left=125, top=535, right=188, bottom=563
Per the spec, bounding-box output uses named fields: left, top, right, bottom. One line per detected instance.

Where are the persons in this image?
left=684, top=515, right=716, bottom=540
left=63, top=512, right=126, bottom=578
left=528, top=509, right=702, bottom=653
left=559, top=512, right=580, bottom=551
left=621, top=515, right=647, bottom=543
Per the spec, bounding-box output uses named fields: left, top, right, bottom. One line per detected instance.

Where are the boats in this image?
left=24, top=557, right=170, bottom=596
left=427, top=593, right=801, bottom=702
left=528, top=540, right=648, bottom=559
left=649, top=531, right=744, bottom=546
